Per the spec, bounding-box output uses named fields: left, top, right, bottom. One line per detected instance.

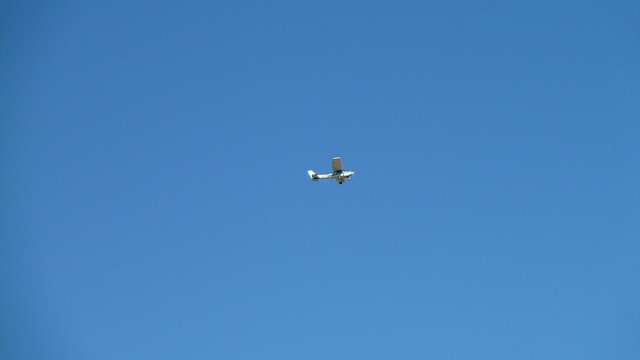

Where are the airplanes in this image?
left=308, top=157, right=356, bottom=184
